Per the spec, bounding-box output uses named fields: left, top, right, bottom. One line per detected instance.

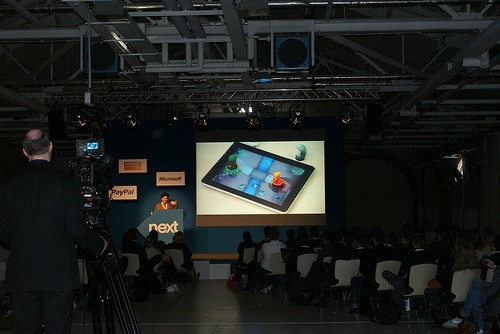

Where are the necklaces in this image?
left=161, top=203, right=168, bottom=209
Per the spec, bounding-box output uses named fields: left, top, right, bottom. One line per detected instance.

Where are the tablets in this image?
left=201, top=142, right=316, bottom=214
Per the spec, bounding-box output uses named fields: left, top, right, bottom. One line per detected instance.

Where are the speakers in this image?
left=275, top=36, right=310, bottom=70
left=85, top=41, right=120, bottom=74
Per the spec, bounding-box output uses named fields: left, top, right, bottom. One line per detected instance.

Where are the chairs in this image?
left=78, top=247, right=500, bottom=334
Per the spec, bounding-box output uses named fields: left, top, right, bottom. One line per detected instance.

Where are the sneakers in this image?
left=443, top=317, right=462, bottom=328
left=477, top=329, right=490, bottom=334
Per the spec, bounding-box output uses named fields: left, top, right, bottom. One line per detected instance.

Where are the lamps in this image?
left=76, top=101, right=354, bottom=128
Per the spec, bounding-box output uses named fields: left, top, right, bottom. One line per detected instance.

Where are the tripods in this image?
left=76, top=184, right=140, bottom=334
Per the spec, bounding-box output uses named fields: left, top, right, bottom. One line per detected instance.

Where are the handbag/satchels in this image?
left=375, top=302, right=404, bottom=324
left=452, top=320, right=476, bottom=334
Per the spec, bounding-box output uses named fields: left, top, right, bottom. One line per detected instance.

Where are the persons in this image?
left=234, top=225, right=500, bottom=334
left=0, top=129, right=109, bottom=334
left=154, top=193, right=178, bottom=212
left=0, top=225, right=201, bottom=316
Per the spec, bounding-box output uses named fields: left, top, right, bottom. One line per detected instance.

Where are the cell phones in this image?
left=481, top=259, right=489, bottom=264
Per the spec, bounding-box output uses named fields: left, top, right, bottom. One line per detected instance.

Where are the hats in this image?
left=487, top=252, right=500, bottom=265
